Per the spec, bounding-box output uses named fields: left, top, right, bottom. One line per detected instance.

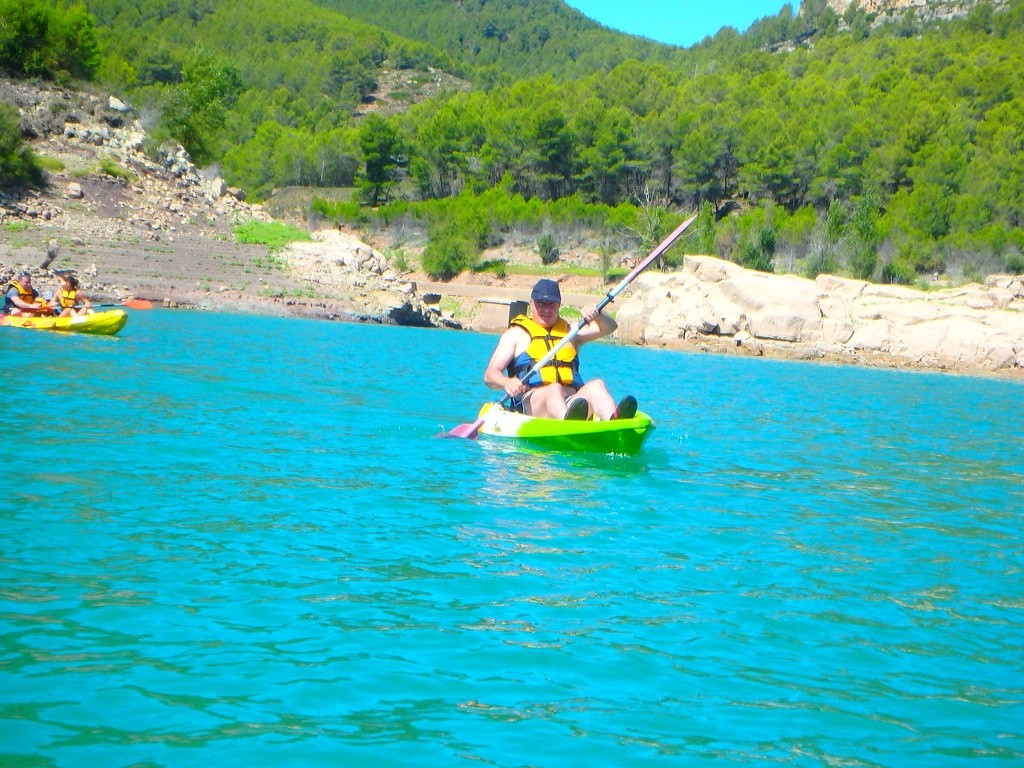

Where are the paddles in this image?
left=0, top=307, right=51, bottom=313
left=438, top=213, right=699, bottom=441
left=51, top=299, right=152, bottom=316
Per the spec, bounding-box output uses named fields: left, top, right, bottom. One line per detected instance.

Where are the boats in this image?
left=476, top=400, right=657, bottom=458
left=0, top=308, right=129, bottom=335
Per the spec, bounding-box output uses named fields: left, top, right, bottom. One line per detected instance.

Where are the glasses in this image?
left=20, top=272, right=30, bottom=276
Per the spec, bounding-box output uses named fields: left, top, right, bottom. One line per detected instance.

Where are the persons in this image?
left=3, top=272, right=93, bottom=317
left=484, top=280, right=637, bottom=420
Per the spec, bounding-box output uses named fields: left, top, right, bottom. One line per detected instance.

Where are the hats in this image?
left=531, top=278, right=561, bottom=303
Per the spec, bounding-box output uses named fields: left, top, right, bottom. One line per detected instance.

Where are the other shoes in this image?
left=564, top=398, right=588, bottom=420
left=610, top=394, right=638, bottom=420
